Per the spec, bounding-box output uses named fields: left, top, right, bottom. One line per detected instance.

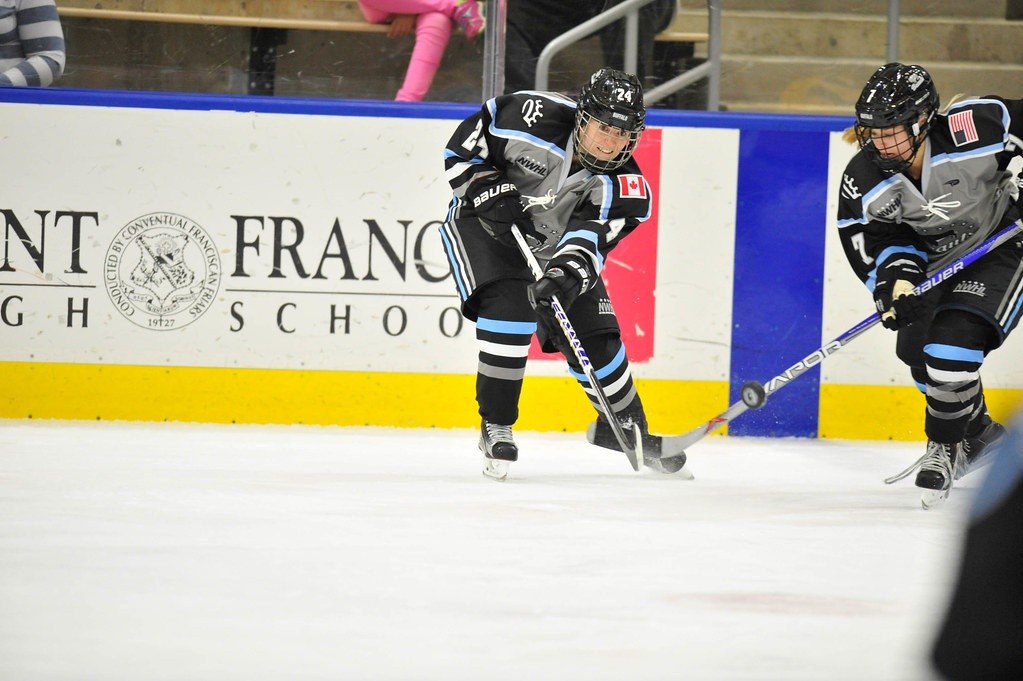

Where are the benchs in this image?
left=51, top=0, right=710, bottom=98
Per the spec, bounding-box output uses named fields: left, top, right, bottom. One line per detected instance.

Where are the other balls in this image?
left=742, top=380, right=767, bottom=410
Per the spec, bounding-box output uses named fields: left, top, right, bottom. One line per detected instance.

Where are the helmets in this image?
left=575, top=67, right=646, bottom=174
left=854, top=62, right=940, bottom=173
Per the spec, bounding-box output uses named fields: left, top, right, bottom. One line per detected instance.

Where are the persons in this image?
left=481, top=0, right=678, bottom=104
left=440, top=66, right=688, bottom=474
left=834, top=60, right=1023, bottom=493
left=357, top=0, right=486, bottom=103
left=1, top=0, right=65, bottom=90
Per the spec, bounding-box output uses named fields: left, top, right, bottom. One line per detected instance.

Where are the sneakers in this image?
left=452, top=0, right=487, bottom=39
left=913, top=439, right=960, bottom=509
left=477, top=418, right=518, bottom=482
left=619, top=415, right=695, bottom=478
left=954, top=414, right=1007, bottom=479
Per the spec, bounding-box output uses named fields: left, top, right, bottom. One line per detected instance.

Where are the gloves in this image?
left=873, top=263, right=927, bottom=330
left=465, top=177, right=548, bottom=248
left=532, top=252, right=593, bottom=328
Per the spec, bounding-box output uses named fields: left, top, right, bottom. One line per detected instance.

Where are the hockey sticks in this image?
left=586, top=217, right=1023, bottom=462
left=511, top=223, right=686, bottom=474
